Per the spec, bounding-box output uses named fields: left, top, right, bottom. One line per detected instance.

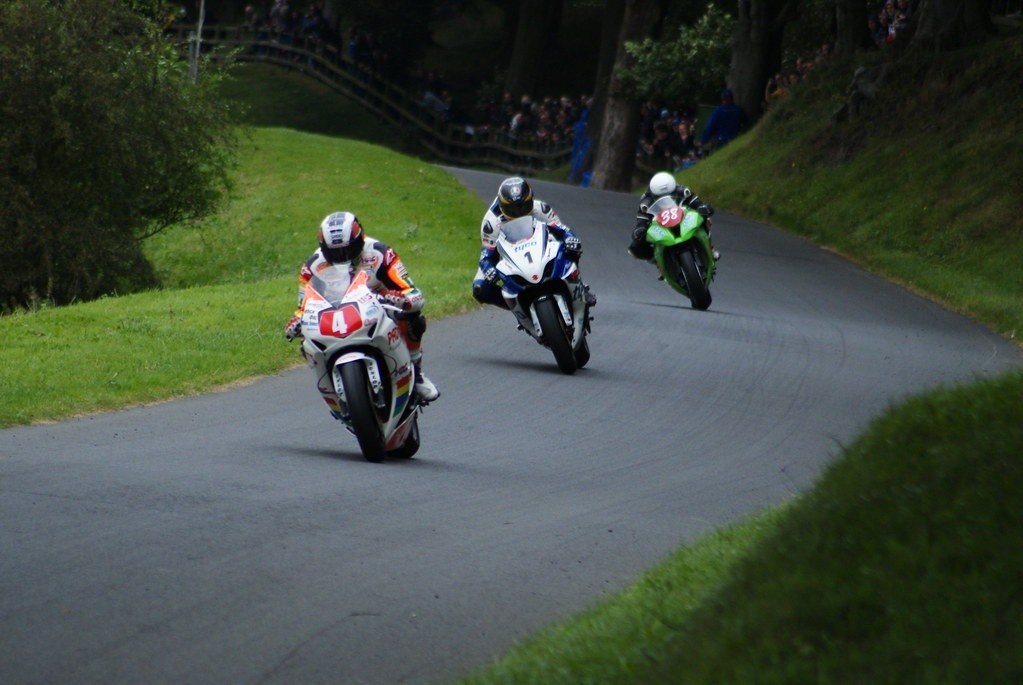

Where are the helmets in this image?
left=649, top=171, right=675, bottom=200
left=497, top=177, right=534, bottom=220
left=319, top=212, right=365, bottom=270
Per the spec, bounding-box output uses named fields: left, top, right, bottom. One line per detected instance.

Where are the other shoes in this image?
left=585, top=292, right=597, bottom=305
left=711, top=248, right=720, bottom=260
left=658, top=273, right=663, bottom=280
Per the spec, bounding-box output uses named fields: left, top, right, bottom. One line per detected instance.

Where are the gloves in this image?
left=636, top=228, right=647, bottom=241
left=486, top=268, right=498, bottom=281
left=698, top=206, right=710, bottom=219
left=286, top=317, right=303, bottom=338
left=564, top=237, right=580, bottom=250
left=385, top=290, right=411, bottom=311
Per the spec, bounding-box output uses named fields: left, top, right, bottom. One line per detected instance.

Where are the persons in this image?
left=765, top=42, right=831, bottom=103
left=503, top=91, right=592, bottom=152
left=285, top=212, right=440, bottom=401
left=472, top=176, right=596, bottom=331
left=636, top=101, right=697, bottom=160
left=696, top=88, right=750, bottom=155
left=347, top=26, right=459, bottom=139
left=245, top=0, right=324, bottom=62
left=867, top=0, right=912, bottom=47
left=628, top=172, right=719, bottom=281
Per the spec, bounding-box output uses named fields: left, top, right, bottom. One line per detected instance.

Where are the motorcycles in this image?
left=636, top=188, right=720, bottom=310
left=484, top=214, right=597, bottom=375
left=285, top=262, right=441, bottom=462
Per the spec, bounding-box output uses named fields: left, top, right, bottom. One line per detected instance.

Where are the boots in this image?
left=413, top=353, right=439, bottom=401
